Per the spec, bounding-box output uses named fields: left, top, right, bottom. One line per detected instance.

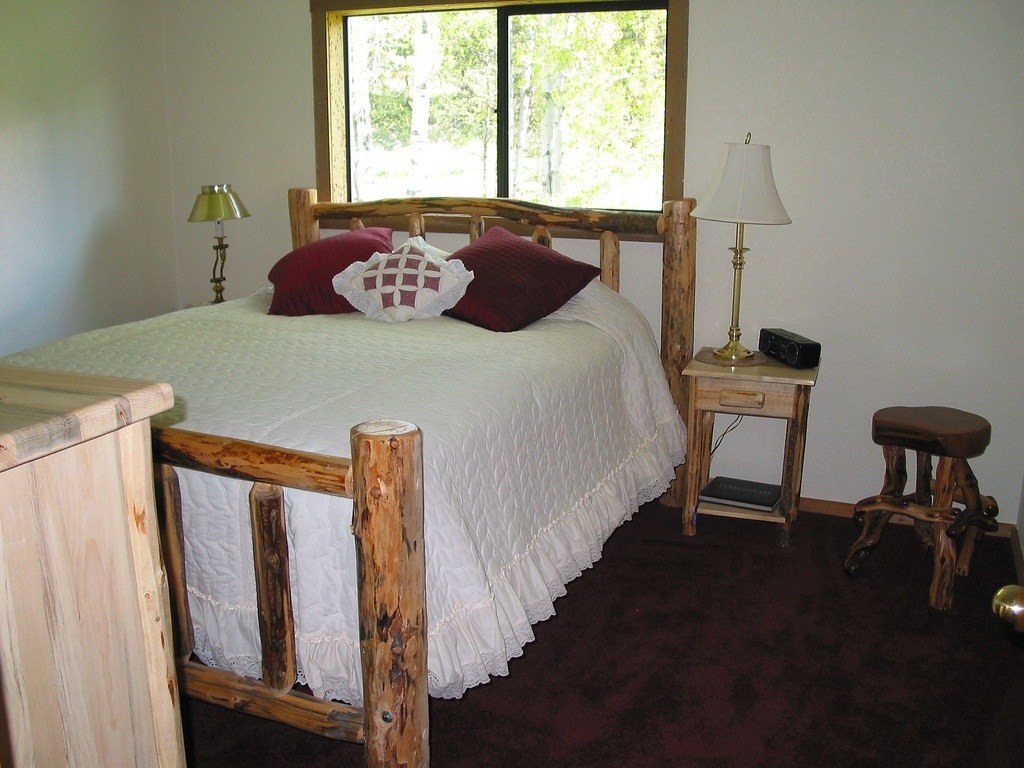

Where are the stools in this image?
left=841, top=406, right=999, bottom=613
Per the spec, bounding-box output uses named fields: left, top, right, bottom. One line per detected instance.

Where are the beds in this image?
left=0, top=188, right=699, bottom=768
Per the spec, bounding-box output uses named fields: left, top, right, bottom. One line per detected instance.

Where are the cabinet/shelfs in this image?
left=2, top=366, right=188, bottom=768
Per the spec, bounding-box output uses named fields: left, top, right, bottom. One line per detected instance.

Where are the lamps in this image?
left=690, top=131, right=791, bottom=368
left=187, top=184, right=252, bottom=304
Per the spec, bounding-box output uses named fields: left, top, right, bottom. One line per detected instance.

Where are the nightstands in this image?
left=681, top=346, right=821, bottom=547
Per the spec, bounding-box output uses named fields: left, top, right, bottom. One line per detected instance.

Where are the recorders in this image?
left=759, top=328, right=821, bottom=369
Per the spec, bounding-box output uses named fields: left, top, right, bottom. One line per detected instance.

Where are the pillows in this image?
left=267, top=227, right=394, bottom=318
left=332, top=235, right=474, bottom=323
left=440, top=226, right=603, bottom=333
